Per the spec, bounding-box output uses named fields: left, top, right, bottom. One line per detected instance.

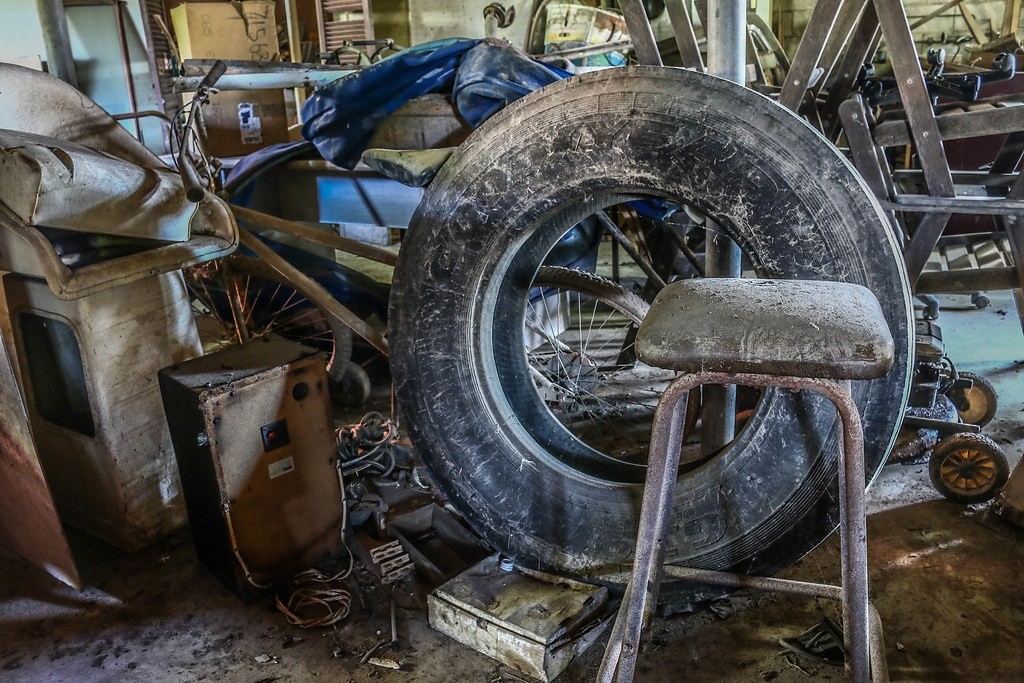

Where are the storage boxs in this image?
left=170, top=0, right=289, bottom=158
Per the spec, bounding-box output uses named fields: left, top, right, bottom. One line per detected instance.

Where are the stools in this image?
left=596, top=278, right=894, bottom=683
